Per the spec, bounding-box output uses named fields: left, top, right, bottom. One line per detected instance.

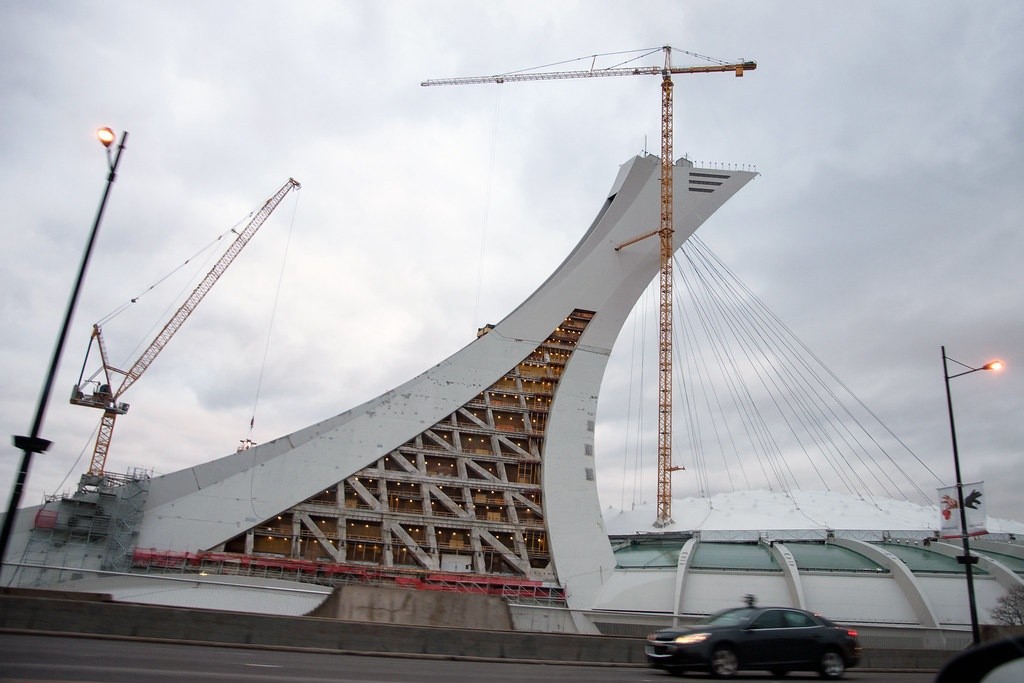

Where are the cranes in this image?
left=421, top=44, right=758, bottom=520
left=69, top=176, right=301, bottom=485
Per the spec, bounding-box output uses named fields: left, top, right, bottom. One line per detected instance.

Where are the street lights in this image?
left=940, top=360, right=1002, bottom=642
left=0, top=128, right=128, bottom=565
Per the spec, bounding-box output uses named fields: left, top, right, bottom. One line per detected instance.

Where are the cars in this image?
left=643, top=606, right=864, bottom=679
